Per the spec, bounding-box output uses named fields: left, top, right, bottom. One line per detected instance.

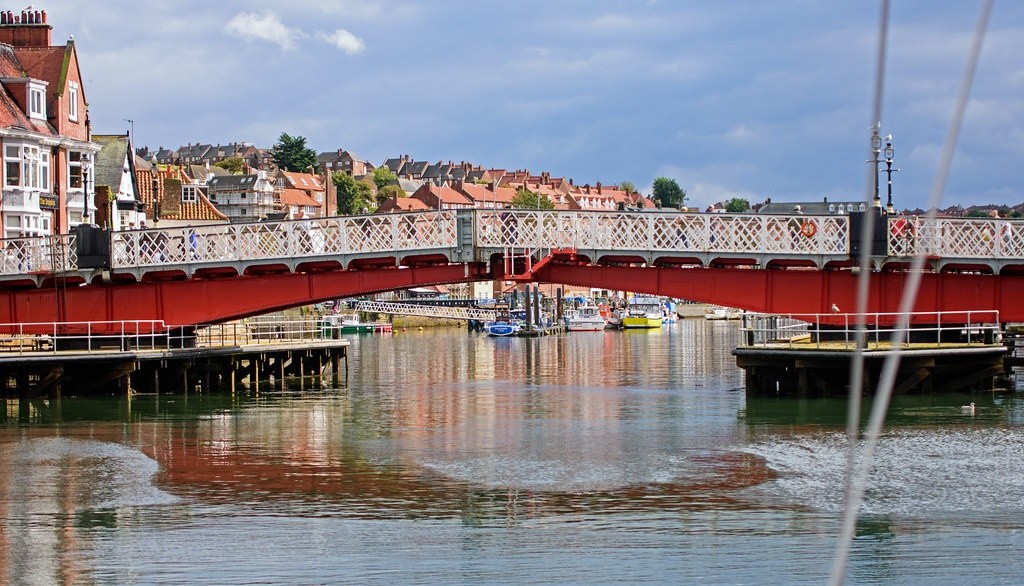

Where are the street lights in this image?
left=150, top=162, right=159, bottom=225
left=879, top=142, right=902, bottom=214
left=865, top=131, right=886, bottom=207
left=78, top=152, right=92, bottom=225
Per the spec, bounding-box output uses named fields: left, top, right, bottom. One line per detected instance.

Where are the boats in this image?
left=662, top=304, right=672, bottom=323
left=666, top=302, right=679, bottom=323
left=566, top=305, right=607, bottom=332
left=704, top=308, right=725, bottom=320
left=622, top=297, right=661, bottom=329
left=726, top=308, right=742, bottom=320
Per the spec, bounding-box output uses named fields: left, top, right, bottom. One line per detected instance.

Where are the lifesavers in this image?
left=802, top=221, right=817, bottom=238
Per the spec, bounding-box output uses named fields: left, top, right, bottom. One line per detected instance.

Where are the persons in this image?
left=330, top=304, right=343, bottom=340
left=358, top=208, right=373, bottom=253
left=124, top=221, right=199, bottom=265
left=654, top=205, right=717, bottom=249
left=280, top=210, right=314, bottom=255
left=500, top=206, right=518, bottom=246
left=611, top=201, right=646, bottom=249
left=405, top=205, right=420, bottom=248
left=785, top=205, right=805, bottom=251
left=985, top=209, right=1016, bottom=255
left=17, top=232, right=41, bottom=274
